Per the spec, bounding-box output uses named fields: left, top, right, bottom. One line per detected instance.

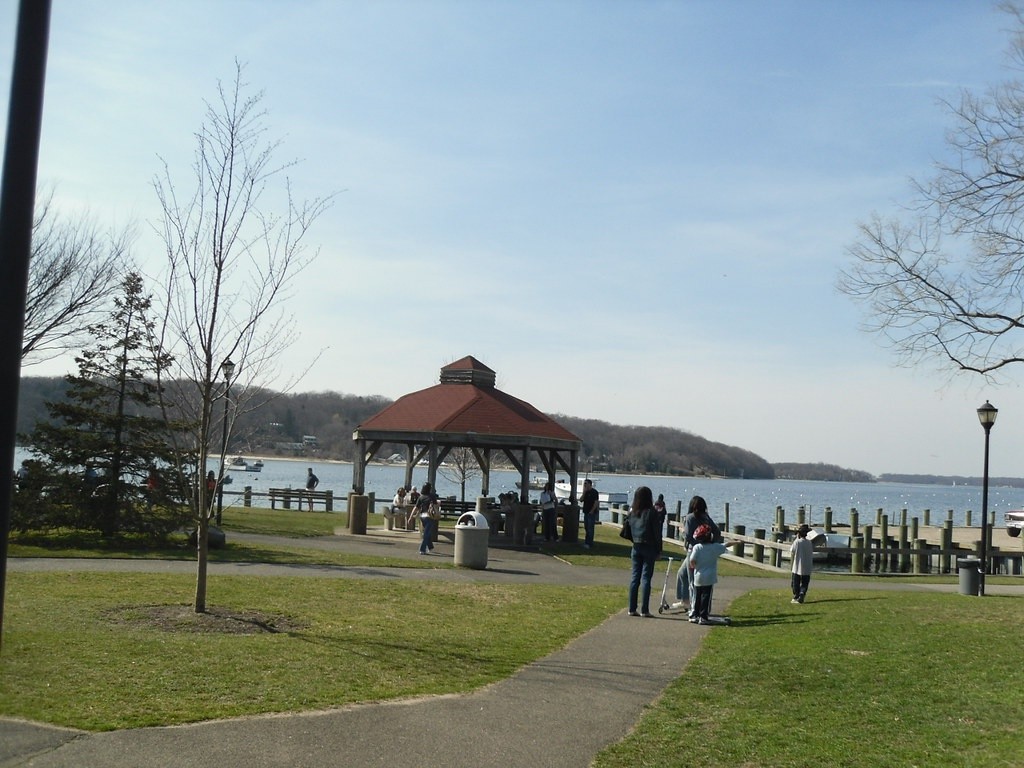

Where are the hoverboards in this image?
left=657, top=558, right=691, bottom=614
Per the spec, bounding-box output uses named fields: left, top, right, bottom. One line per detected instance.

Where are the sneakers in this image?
left=689, top=616, right=711, bottom=624
left=673, top=601, right=694, bottom=615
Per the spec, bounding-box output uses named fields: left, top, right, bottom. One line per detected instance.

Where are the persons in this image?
left=580, top=479, right=599, bottom=549
left=790, top=524, right=813, bottom=604
left=305, top=468, right=319, bottom=512
left=540, top=483, right=560, bottom=543
left=393, top=486, right=420, bottom=529
left=408, top=485, right=438, bottom=554
left=628, top=486, right=666, bottom=617
left=207, top=471, right=218, bottom=518
left=17, top=462, right=29, bottom=489
left=688, top=525, right=741, bottom=624
left=83, top=461, right=113, bottom=495
left=672, top=496, right=721, bottom=616
left=144, top=464, right=161, bottom=510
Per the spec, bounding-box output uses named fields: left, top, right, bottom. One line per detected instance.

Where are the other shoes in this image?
left=799, top=592, right=804, bottom=603
left=640, top=613, right=654, bottom=617
left=628, top=611, right=640, bottom=616
left=791, top=599, right=799, bottom=603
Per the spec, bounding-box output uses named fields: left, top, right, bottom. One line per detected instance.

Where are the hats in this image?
left=412, top=486, right=417, bottom=490
left=799, top=525, right=809, bottom=536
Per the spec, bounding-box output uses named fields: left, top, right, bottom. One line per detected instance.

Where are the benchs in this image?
left=137, top=481, right=199, bottom=501
left=438, top=500, right=542, bottom=520
left=269, top=487, right=334, bottom=511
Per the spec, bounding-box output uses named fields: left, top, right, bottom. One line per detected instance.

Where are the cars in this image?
left=1004, top=506, right=1024, bottom=537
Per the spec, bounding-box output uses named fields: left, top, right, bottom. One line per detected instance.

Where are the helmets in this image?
left=693, top=524, right=711, bottom=540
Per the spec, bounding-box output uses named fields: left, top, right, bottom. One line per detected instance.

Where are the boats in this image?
left=224, top=456, right=266, bottom=472
left=554, top=476, right=629, bottom=508
left=205, top=474, right=233, bottom=485
left=514, top=477, right=549, bottom=489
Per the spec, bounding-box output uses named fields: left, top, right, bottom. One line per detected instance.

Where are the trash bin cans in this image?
left=957, top=558, right=980, bottom=595
left=453, top=511, right=490, bottom=570
left=349, top=496, right=369, bottom=536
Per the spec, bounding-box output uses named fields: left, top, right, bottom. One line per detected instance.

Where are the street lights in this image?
left=976, top=400, right=999, bottom=596
left=218, top=358, right=235, bottom=523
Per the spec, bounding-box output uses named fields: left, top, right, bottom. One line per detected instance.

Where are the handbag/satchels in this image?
left=620, top=519, right=634, bottom=544
left=428, top=501, right=441, bottom=520
